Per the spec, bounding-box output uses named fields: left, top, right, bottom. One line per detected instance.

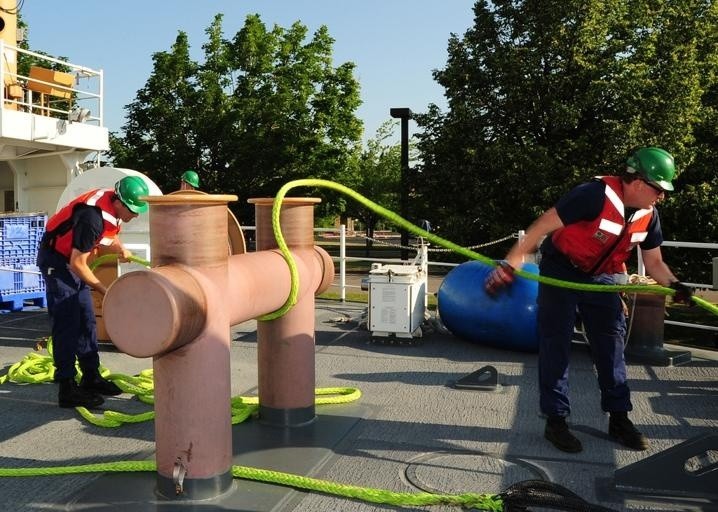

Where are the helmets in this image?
left=627, top=147, right=676, bottom=191
left=181, top=171, right=200, bottom=188
left=115, top=175, right=149, bottom=214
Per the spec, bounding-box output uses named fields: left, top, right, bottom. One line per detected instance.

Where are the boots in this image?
left=544, top=414, right=582, bottom=453
left=59, top=377, right=103, bottom=409
left=609, top=411, right=649, bottom=450
left=78, top=367, right=123, bottom=395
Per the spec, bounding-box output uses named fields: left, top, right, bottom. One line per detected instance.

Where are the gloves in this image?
left=668, top=282, right=697, bottom=309
left=483, top=262, right=514, bottom=296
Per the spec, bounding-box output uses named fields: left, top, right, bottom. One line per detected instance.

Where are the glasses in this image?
left=640, top=178, right=664, bottom=196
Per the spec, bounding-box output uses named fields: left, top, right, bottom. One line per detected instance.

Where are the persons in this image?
left=179, top=171, right=200, bottom=192
left=37, top=175, right=148, bottom=410
left=485, top=148, right=696, bottom=456
